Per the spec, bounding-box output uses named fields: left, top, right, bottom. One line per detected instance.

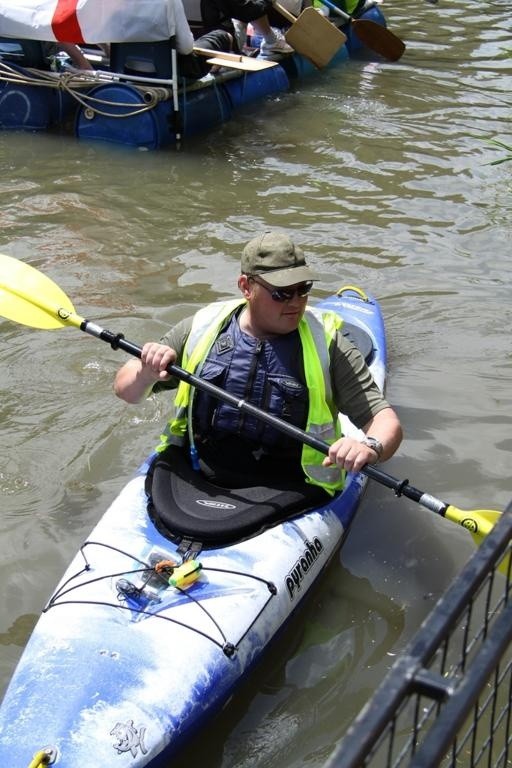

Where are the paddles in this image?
left=2, top=257, right=512, bottom=578
left=271, top=0, right=347, bottom=69
left=193, top=47, right=279, bottom=72
left=319, top=0, right=406, bottom=62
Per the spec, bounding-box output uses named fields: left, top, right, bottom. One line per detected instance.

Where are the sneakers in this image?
left=260, top=36, right=295, bottom=54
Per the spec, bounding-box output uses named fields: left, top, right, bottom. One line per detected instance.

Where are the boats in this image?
left=1, top=286, right=388, bottom=768
left=1, top=1, right=386, bottom=148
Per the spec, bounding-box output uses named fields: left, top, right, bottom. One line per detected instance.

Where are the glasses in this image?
left=253, top=279, right=313, bottom=302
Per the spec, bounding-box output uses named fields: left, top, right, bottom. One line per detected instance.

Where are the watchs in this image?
left=363, top=436, right=383, bottom=466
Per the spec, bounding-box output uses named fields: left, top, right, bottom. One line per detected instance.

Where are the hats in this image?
left=241, top=231, right=320, bottom=288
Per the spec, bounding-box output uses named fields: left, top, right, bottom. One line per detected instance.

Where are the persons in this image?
left=114, top=231, right=403, bottom=501
left=220, top=1, right=295, bottom=53
left=314, top=0, right=381, bottom=17
left=106, top=0, right=233, bottom=79
